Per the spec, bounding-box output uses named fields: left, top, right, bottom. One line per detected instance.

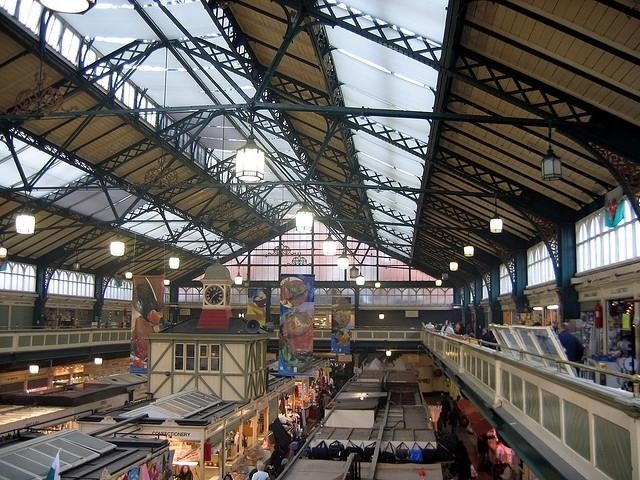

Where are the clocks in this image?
left=203, top=284, right=226, bottom=307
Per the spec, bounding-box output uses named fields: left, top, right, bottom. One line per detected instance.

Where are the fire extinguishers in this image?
left=205, top=438, right=212, bottom=460
left=594, top=306, right=603, bottom=329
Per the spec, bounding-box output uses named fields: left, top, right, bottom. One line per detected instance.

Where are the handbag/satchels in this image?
left=307, top=440, right=450, bottom=463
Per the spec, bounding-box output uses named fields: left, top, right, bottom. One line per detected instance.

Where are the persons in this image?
left=251, top=441, right=294, bottom=480
left=422, top=320, right=585, bottom=362
left=428, top=392, right=512, bottom=480
left=179, top=465, right=193, bottom=480
left=223, top=474, right=233, bottom=480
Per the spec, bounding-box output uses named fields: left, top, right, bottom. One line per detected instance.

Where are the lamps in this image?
left=539, top=121, right=563, bottom=182
left=295, top=182, right=365, bottom=287
left=450, top=196, right=504, bottom=271
left=233, top=107, right=266, bottom=185
